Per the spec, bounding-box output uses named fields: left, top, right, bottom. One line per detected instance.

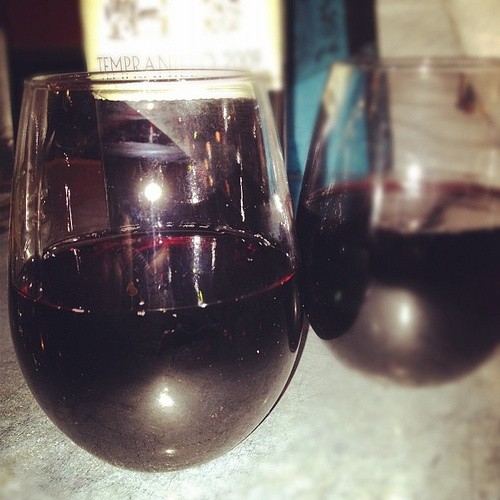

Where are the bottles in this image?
left=75, top=0, right=290, bottom=247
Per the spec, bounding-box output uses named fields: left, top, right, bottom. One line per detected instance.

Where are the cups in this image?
left=7, top=68, right=313, bottom=473
left=291, top=53, right=500, bottom=385
